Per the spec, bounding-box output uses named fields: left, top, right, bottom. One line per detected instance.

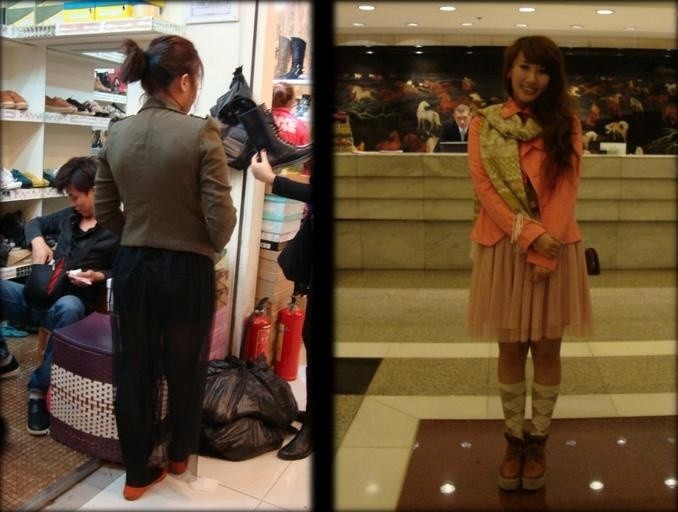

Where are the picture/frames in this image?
left=182, top=1, right=243, bottom=25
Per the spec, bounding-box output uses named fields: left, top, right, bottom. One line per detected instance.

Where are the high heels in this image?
left=26, top=390, right=52, bottom=436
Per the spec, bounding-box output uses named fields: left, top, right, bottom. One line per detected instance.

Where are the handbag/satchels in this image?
left=584, top=245, right=601, bottom=277
left=23, top=253, right=83, bottom=313
left=209, top=64, right=257, bottom=127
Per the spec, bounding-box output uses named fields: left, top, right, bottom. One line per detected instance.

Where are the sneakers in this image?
left=0, top=89, right=29, bottom=110
left=0, top=355, right=22, bottom=381
left=45, top=94, right=126, bottom=120
left=95, top=69, right=118, bottom=93
left=0, top=166, right=58, bottom=191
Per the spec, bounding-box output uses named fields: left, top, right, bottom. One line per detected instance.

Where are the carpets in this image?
left=0, top=327, right=104, bottom=512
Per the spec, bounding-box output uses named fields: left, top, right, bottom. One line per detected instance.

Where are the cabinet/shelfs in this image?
left=90, top=60, right=126, bottom=159
left=272, top=76, right=312, bottom=126
left=1, top=37, right=111, bottom=283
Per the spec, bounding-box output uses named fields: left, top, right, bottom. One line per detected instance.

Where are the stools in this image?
left=49, top=311, right=173, bottom=466
left=34, top=324, right=52, bottom=365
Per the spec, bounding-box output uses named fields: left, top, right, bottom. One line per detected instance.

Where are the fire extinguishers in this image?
left=239, top=297, right=273, bottom=370
left=273, top=294, right=304, bottom=381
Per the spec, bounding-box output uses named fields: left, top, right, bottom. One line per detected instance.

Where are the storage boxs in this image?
left=253, top=173, right=311, bottom=372
left=1, top=1, right=167, bottom=27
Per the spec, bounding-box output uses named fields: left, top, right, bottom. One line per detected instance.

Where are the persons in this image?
left=442, top=104, right=473, bottom=148
left=0, top=153, right=118, bottom=439
left=94, top=37, right=241, bottom=499
left=274, top=83, right=309, bottom=172
left=250, top=147, right=313, bottom=461
left=466, top=36, right=595, bottom=493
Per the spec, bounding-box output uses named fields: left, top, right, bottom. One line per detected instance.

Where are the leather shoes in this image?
left=122, top=462, right=168, bottom=501
left=168, top=451, right=190, bottom=475
left=279, top=409, right=315, bottom=460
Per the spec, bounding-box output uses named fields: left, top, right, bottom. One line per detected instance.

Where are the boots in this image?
left=274, top=32, right=307, bottom=79
left=242, top=104, right=312, bottom=170
left=498, top=433, right=550, bottom=490
